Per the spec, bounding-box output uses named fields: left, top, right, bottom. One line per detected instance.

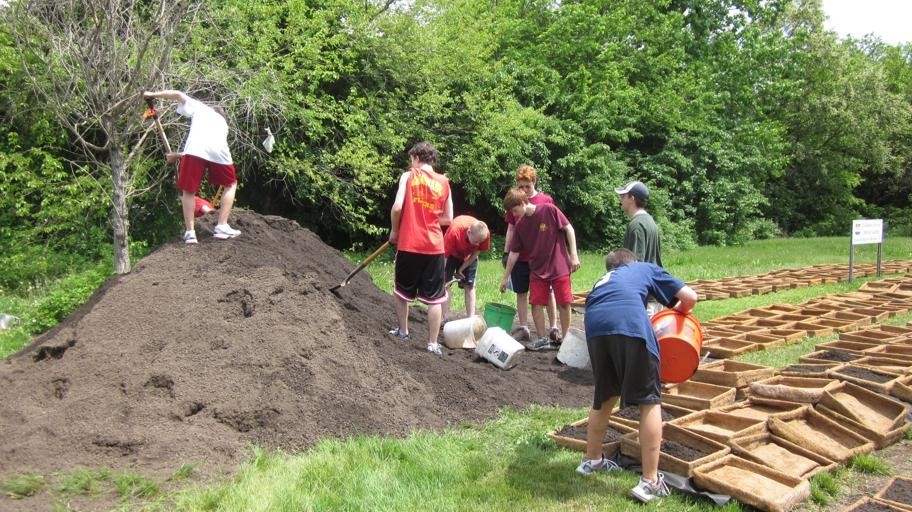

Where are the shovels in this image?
left=328, top=240, right=393, bottom=291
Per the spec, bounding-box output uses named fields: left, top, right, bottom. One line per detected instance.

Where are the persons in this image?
left=502, top=166, right=560, bottom=341
left=388, top=142, right=455, bottom=355
left=443, top=214, right=491, bottom=328
left=576, top=248, right=697, bottom=505
left=500, top=188, right=580, bottom=351
left=143, top=90, right=242, bottom=245
left=613, top=181, right=662, bottom=319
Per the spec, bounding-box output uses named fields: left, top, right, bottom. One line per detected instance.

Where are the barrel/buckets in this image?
left=483, top=290, right=519, bottom=332
left=442, top=314, right=489, bottom=349
left=556, top=328, right=591, bottom=369
left=474, top=326, right=527, bottom=371
left=650, top=307, right=711, bottom=384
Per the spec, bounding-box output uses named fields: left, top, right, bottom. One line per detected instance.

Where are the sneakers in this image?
left=575, top=453, right=622, bottom=475
left=184, top=230, right=197, bottom=244
left=510, top=326, right=563, bottom=351
left=614, top=180, right=649, bottom=202
left=631, top=473, right=670, bottom=503
left=213, top=224, right=241, bottom=239
left=428, top=342, right=442, bottom=355
left=389, top=328, right=409, bottom=341
